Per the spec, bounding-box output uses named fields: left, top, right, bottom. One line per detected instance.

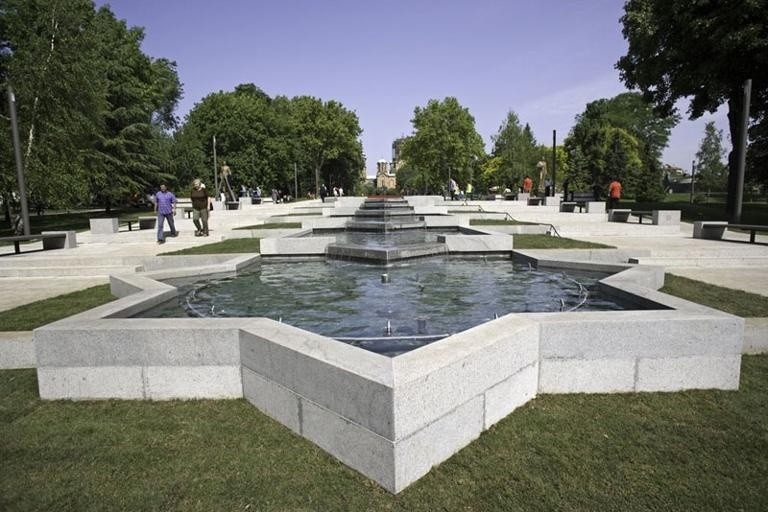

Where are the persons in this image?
left=320, top=183, right=345, bottom=203
left=523, top=175, right=532, bottom=194
left=191, top=178, right=210, bottom=236
left=153, top=183, right=177, bottom=245
left=536, top=155, right=547, bottom=190
left=219, top=160, right=231, bottom=192
left=441, top=177, right=475, bottom=202
left=606, top=177, right=622, bottom=209
left=240, top=184, right=292, bottom=204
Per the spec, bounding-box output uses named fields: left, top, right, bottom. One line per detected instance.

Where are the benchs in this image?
left=0, top=229, right=78, bottom=254
left=692, top=220, right=767, bottom=244
left=89, top=196, right=274, bottom=235
left=495, top=192, right=682, bottom=226
